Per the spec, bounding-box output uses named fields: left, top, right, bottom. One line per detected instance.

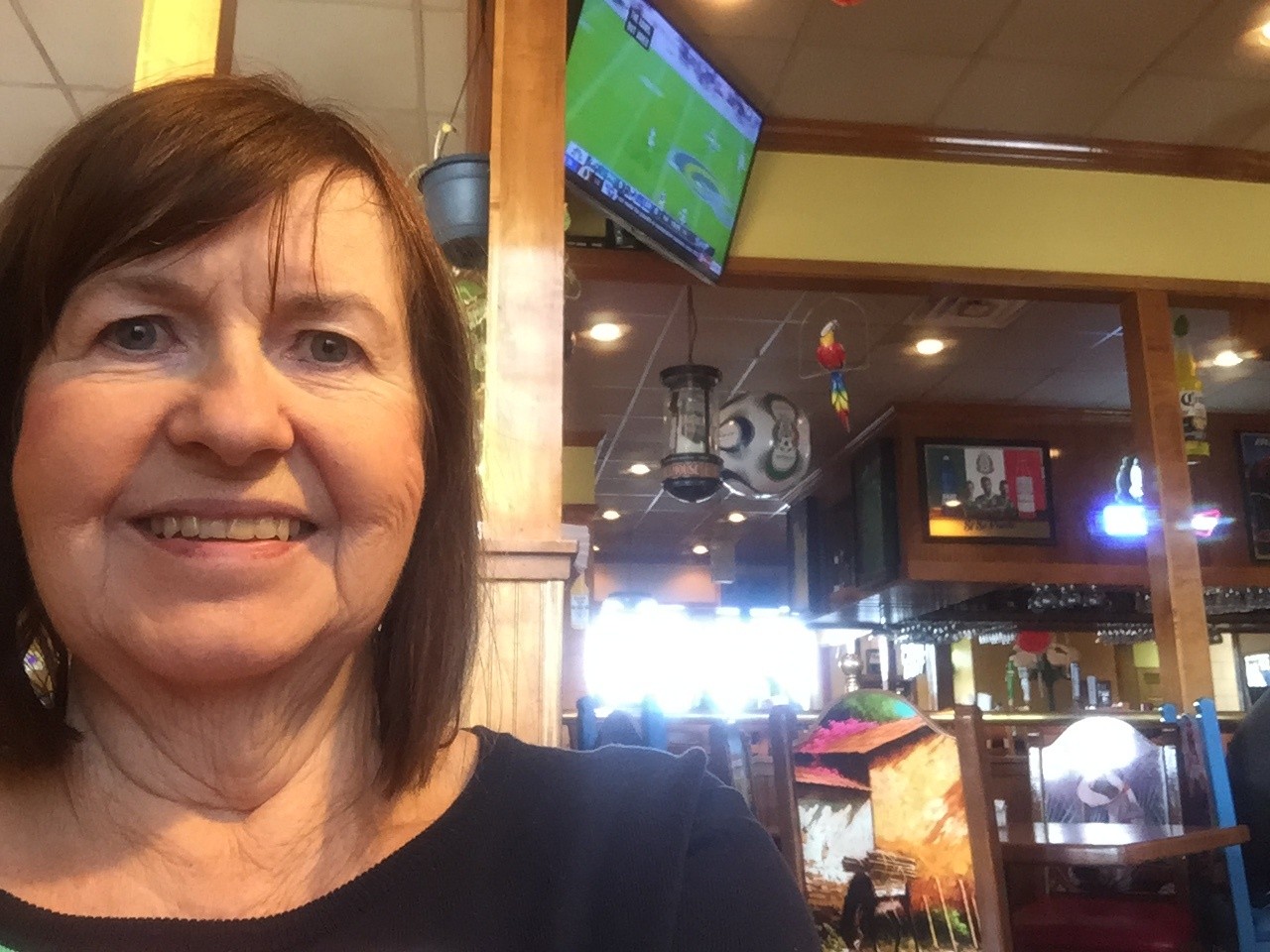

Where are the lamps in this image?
left=659, top=285, right=729, bottom=506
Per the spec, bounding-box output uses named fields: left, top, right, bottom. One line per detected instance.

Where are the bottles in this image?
left=1170, top=328, right=1211, bottom=463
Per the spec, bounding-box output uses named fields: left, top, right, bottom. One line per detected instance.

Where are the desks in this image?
left=999, top=821, right=1251, bottom=866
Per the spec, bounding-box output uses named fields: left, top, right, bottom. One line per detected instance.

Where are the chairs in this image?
left=1011, top=715, right=1196, bottom=952
left=1158, top=697, right=1270, bottom=952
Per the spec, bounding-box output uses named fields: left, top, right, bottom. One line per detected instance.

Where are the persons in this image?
left=1191, top=687, right=1270, bottom=952
left=0, top=53, right=822, bottom=952
left=967, top=476, right=1015, bottom=510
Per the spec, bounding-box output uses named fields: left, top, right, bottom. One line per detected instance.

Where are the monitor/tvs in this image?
left=567, top=0, right=767, bottom=283
